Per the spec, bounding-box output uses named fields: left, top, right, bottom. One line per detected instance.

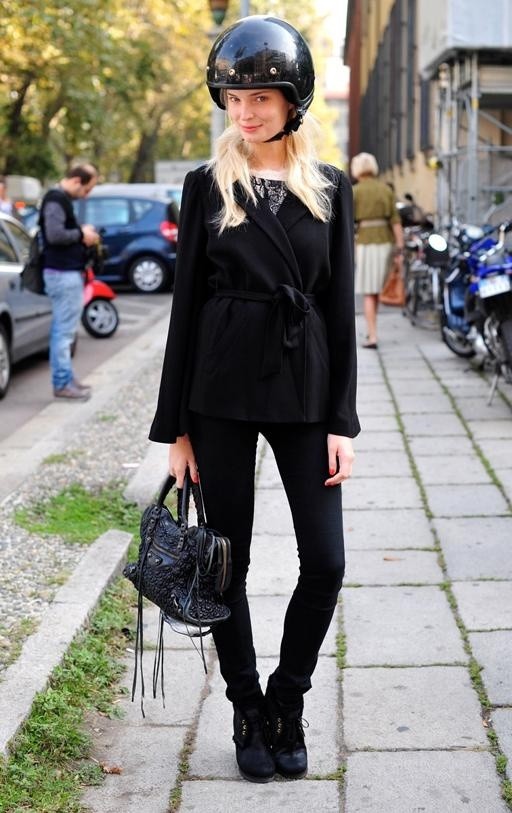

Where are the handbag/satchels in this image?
left=19, top=231, right=46, bottom=295
left=377, top=257, right=408, bottom=305
left=123, top=465, right=234, bottom=625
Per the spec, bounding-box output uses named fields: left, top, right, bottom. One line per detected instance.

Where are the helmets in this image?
left=205, top=16, right=316, bottom=115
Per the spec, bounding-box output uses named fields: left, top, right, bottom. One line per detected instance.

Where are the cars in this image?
left=1, top=212, right=80, bottom=402
left=20, top=178, right=182, bottom=298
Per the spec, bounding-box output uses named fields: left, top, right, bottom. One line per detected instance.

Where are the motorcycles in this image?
left=388, top=182, right=511, bottom=408
left=71, top=222, right=122, bottom=342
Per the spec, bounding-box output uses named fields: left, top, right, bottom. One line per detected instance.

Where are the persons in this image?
left=40, top=162, right=106, bottom=400
left=0, top=178, right=20, bottom=222
left=147, top=16, right=362, bottom=779
left=395, top=192, right=424, bottom=226
left=350, top=150, right=405, bottom=348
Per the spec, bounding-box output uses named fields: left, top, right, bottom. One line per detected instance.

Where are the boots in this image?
left=264, top=681, right=312, bottom=780
left=227, top=681, right=275, bottom=782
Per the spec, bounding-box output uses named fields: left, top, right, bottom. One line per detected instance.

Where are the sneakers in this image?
left=52, top=379, right=89, bottom=401
